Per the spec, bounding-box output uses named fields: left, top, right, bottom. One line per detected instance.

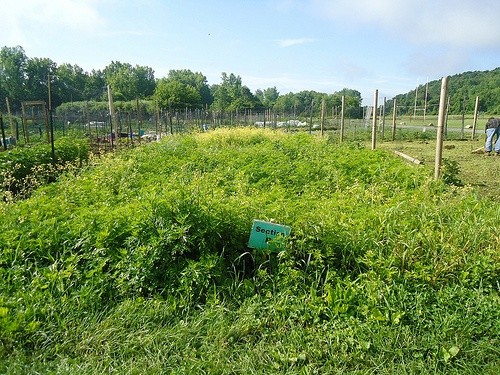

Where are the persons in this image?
left=485, top=116, right=500, bottom=156
